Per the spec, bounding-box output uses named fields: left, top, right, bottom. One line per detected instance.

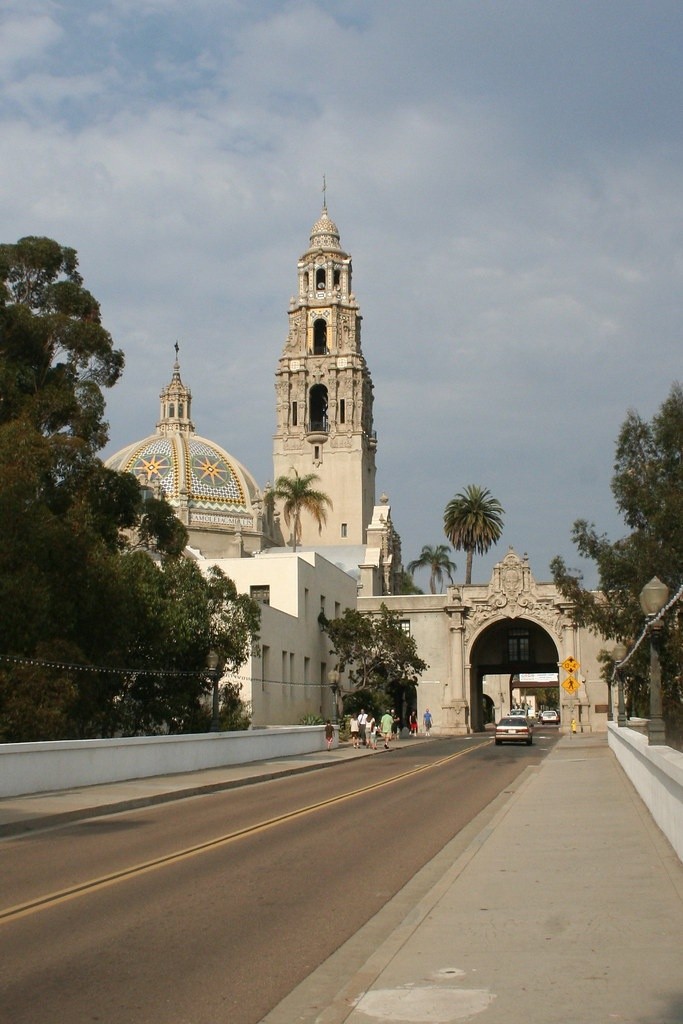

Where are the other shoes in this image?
left=354, top=744, right=378, bottom=751
left=327, top=747, right=333, bottom=751
left=410, top=731, right=416, bottom=737
left=384, top=743, right=388, bottom=749
left=426, top=732, right=430, bottom=736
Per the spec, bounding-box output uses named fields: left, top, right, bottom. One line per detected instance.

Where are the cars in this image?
left=541, top=711, right=559, bottom=725
left=495, top=717, right=534, bottom=746
left=507, top=709, right=527, bottom=718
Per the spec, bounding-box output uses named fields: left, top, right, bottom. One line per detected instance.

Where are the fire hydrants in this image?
left=571, top=720, right=577, bottom=734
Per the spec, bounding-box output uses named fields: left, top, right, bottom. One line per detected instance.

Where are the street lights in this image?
left=638, top=576, right=670, bottom=747
left=328, top=669, right=340, bottom=725
left=613, top=641, right=627, bottom=727
left=207, top=649, right=220, bottom=732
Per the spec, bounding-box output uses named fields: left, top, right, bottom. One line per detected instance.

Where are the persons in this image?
left=381, top=709, right=394, bottom=750
left=369, top=718, right=380, bottom=750
left=364, top=714, right=374, bottom=749
left=391, top=709, right=400, bottom=740
left=325, top=719, right=335, bottom=751
left=350, top=713, right=360, bottom=749
left=358, top=710, right=368, bottom=745
left=424, top=708, right=432, bottom=738
left=409, top=710, right=418, bottom=738
